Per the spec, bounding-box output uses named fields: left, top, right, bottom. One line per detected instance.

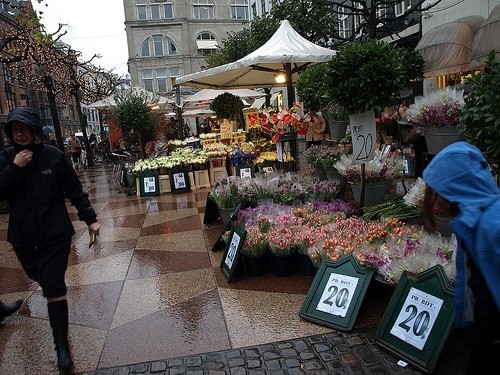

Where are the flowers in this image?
left=128, top=93, right=461, bottom=285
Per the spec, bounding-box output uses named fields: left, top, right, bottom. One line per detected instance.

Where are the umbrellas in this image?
left=86, top=86, right=175, bottom=110
left=42, top=126, right=55, bottom=134
left=176, top=20, right=339, bottom=134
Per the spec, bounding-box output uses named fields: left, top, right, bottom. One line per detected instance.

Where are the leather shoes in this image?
left=0, top=299, right=23, bottom=323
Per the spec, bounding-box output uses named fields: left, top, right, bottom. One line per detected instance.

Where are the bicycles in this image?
left=80, top=141, right=112, bottom=168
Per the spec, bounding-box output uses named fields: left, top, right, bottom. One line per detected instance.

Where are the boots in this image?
left=47, top=299, right=73, bottom=373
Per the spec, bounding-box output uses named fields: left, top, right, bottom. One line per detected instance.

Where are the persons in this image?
left=68, top=133, right=81, bottom=169
left=423, top=142, right=500, bottom=375
left=0, top=107, right=101, bottom=375
left=0, top=299, right=23, bottom=322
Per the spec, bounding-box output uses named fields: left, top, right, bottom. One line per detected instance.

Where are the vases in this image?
left=241, top=251, right=267, bottom=277
left=269, top=248, right=297, bottom=277
left=296, top=250, right=310, bottom=276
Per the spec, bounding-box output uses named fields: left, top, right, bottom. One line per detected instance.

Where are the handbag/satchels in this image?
left=74, top=143, right=81, bottom=150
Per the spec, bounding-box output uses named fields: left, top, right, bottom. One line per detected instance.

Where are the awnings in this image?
left=196, top=41, right=218, bottom=49
left=416, top=14, right=486, bottom=77
left=470, top=4, right=500, bottom=70
